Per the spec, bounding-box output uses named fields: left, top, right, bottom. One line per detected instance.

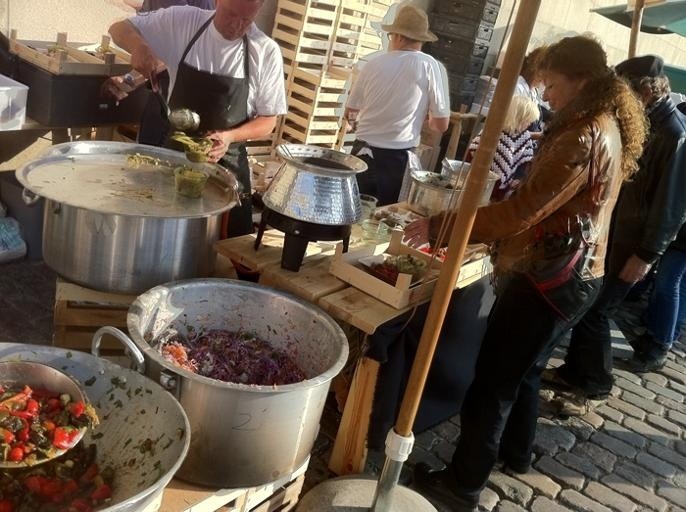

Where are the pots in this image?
left=261, top=142, right=369, bottom=227
left=15, top=140, right=238, bottom=296
left=0, top=325, right=191, bottom=512
left=440, top=158, right=499, bottom=207
left=407, top=170, right=463, bottom=218
left=125, top=277, right=351, bottom=487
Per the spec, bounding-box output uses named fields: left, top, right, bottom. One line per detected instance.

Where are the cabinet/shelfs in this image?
left=269, top=1, right=399, bottom=156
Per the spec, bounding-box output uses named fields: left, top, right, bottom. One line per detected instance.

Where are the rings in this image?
left=417, top=234, right=420, bottom=239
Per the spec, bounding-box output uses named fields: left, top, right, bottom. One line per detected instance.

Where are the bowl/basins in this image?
left=186, top=137, right=213, bottom=163
left=173, top=164, right=210, bottom=199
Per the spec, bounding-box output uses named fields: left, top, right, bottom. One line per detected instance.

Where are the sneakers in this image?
left=540, top=368, right=613, bottom=417
left=411, top=462, right=478, bottom=512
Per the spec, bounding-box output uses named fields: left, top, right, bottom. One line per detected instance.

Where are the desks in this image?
left=217, top=200, right=498, bottom=479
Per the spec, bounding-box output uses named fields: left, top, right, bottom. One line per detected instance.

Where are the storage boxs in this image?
left=0, top=69, right=31, bottom=131
left=9, top=52, right=146, bottom=128
left=0, top=171, right=44, bottom=263
left=428, top=0, right=505, bottom=115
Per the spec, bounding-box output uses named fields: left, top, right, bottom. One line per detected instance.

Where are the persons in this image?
left=403, top=37, right=651, bottom=512
left=108, top=0, right=287, bottom=280
left=343, top=7, right=451, bottom=206
left=469, top=46, right=686, bottom=400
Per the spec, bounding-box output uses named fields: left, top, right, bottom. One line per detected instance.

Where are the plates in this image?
left=360, top=253, right=423, bottom=287
left=418, top=244, right=470, bottom=265
left=0, top=361, right=89, bottom=473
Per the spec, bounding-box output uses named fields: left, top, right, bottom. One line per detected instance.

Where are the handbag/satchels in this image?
left=514, top=208, right=600, bottom=323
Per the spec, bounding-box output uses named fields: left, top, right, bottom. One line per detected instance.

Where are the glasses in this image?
left=386, top=33, right=399, bottom=41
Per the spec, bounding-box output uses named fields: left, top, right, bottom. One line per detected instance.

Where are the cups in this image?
left=361, top=216, right=388, bottom=243
left=355, top=194, right=378, bottom=223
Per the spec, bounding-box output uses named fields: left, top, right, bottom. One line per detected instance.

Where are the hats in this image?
left=381, top=4, right=440, bottom=43
left=616, top=55, right=663, bottom=77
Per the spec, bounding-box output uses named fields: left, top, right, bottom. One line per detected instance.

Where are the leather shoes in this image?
left=622, top=349, right=668, bottom=373
